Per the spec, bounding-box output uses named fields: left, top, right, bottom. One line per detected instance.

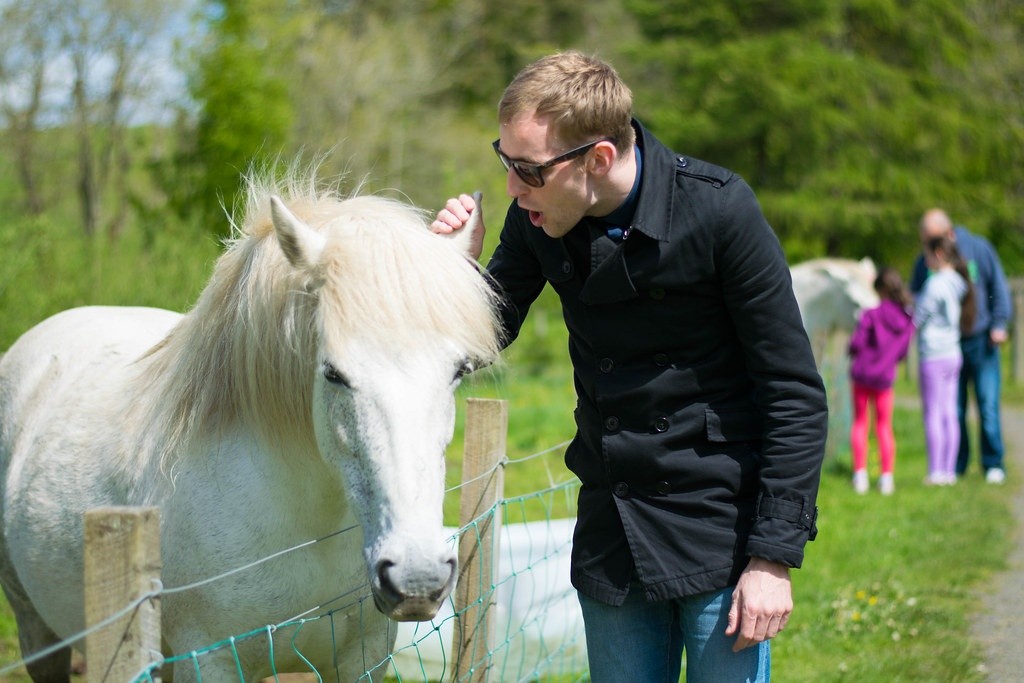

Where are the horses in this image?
left=789, top=254, right=880, bottom=367
left=382, top=514, right=589, bottom=683
left=0, top=132, right=513, bottom=683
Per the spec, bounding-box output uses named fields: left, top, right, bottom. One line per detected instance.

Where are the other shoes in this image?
left=877, top=474, right=895, bottom=495
left=985, top=467, right=1006, bottom=486
left=922, top=471, right=958, bottom=487
left=853, top=469, right=870, bottom=495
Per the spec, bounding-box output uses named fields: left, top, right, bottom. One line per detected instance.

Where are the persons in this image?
left=430, top=51, right=827, bottom=683
left=910, top=207, right=1011, bottom=483
left=847, top=268, right=915, bottom=494
left=912, top=236, right=978, bottom=486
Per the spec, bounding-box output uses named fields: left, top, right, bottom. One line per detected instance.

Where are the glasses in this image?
left=493, top=136, right=617, bottom=187
